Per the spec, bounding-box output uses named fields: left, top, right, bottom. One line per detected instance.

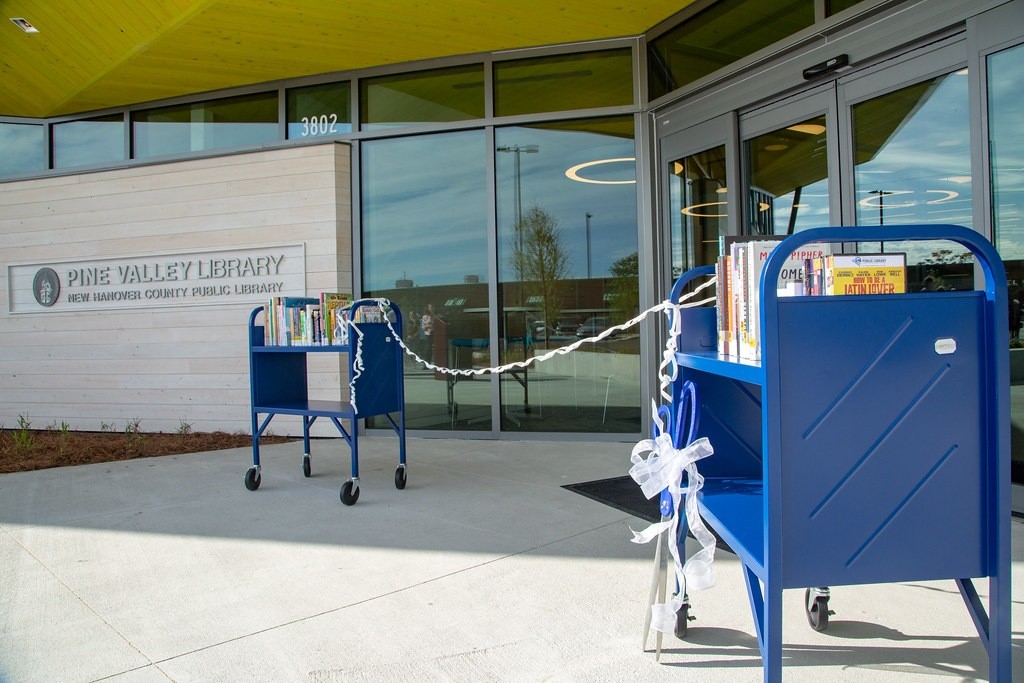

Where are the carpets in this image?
left=559, top=473, right=737, bottom=554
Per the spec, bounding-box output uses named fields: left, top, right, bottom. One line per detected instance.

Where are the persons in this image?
left=1008, top=279, right=1024, bottom=342
left=920, top=276, right=935, bottom=292
left=828, top=270, right=834, bottom=285
left=406, top=304, right=436, bottom=368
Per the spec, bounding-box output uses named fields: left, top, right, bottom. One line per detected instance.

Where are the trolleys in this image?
left=652, top=220, right=1015, bottom=683
left=244, top=297, right=408, bottom=505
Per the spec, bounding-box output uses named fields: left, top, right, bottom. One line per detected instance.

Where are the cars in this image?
left=555, top=316, right=585, bottom=336
left=534, top=320, right=553, bottom=337
left=576, top=316, right=616, bottom=340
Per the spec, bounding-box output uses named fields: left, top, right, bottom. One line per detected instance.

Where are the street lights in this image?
left=585, top=212, right=592, bottom=280
left=868, top=189, right=893, bottom=254
left=496, top=143, right=540, bottom=307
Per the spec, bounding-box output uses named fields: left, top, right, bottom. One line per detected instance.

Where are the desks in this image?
left=466, top=307, right=545, bottom=425
left=561, top=309, right=625, bottom=412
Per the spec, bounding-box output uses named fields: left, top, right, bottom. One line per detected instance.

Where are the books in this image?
left=714, top=234, right=907, bottom=363
left=264, top=292, right=382, bottom=346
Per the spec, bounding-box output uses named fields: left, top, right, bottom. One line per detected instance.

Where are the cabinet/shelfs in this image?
left=661, top=223, right=1012, bottom=683
left=245, top=299, right=407, bottom=507
left=450, top=336, right=530, bottom=421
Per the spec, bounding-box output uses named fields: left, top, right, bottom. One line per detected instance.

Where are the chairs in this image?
left=599, top=349, right=641, bottom=425
